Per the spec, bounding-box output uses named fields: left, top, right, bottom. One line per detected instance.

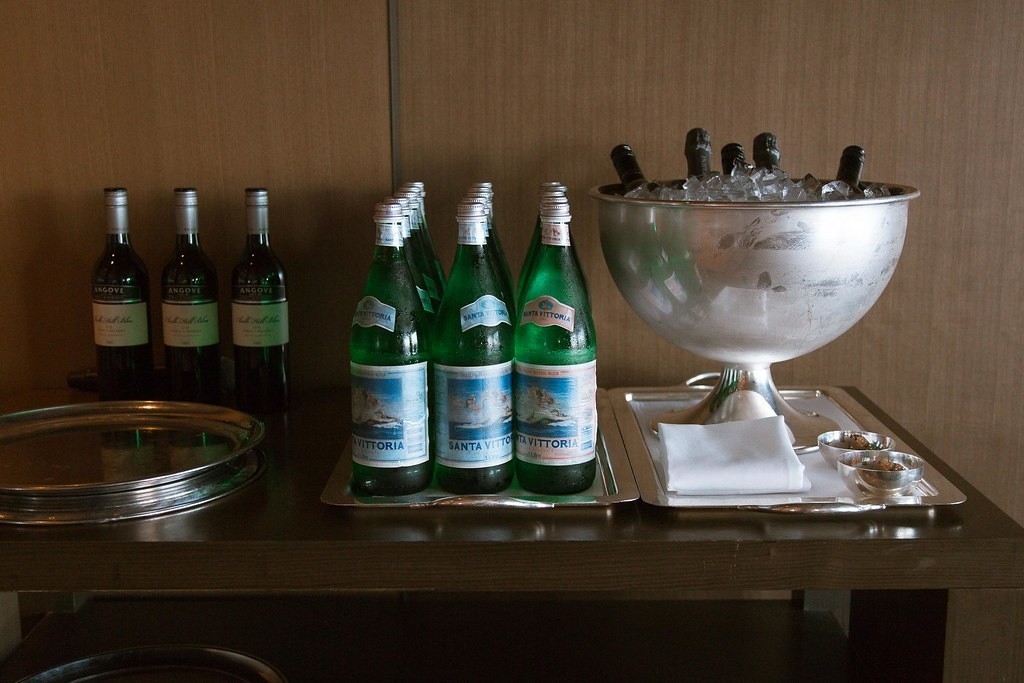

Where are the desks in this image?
left=0, top=378, right=1024, bottom=683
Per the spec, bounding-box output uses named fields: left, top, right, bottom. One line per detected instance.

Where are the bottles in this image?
left=91, top=186, right=155, bottom=400
left=159, top=187, right=223, bottom=404
left=835, top=145, right=866, bottom=186
left=230, top=187, right=292, bottom=417
left=349, top=180, right=599, bottom=495
left=752, top=132, right=782, bottom=171
left=66, top=367, right=98, bottom=390
left=608, top=143, right=652, bottom=195
left=684, top=128, right=713, bottom=182
left=721, top=143, right=746, bottom=175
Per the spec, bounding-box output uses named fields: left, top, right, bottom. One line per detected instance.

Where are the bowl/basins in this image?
left=817, top=431, right=896, bottom=470
left=836, top=450, right=925, bottom=495
left=15, top=643, right=289, bottom=683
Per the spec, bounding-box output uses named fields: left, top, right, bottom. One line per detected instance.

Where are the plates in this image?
left=0, top=398, right=269, bottom=525
